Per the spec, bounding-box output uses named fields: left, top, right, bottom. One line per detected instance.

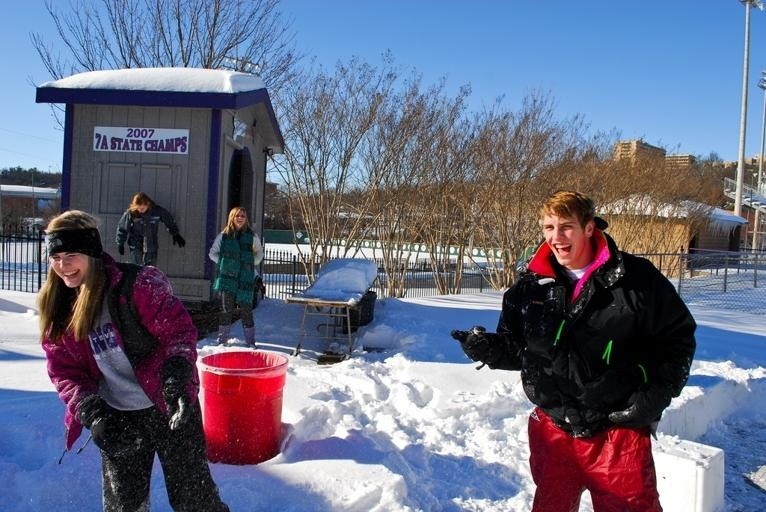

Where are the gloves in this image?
left=78, top=396, right=142, bottom=461
left=450, top=326, right=496, bottom=370
left=172, top=233, right=186, bottom=248
left=161, top=355, right=198, bottom=431
left=608, top=384, right=672, bottom=428
left=118, top=245, right=125, bottom=255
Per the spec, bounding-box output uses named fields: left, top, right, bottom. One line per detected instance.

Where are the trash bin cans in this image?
left=343, top=291, right=377, bottom=334
left=195, top=350, right=289, bottom=466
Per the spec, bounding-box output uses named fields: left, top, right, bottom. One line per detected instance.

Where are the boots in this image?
left=244, top=327, right=257, bottom=349
left=218, top=325, right=230, bottom=346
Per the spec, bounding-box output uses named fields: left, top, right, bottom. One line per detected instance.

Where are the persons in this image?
left=115, top=191, right=186, bottom=266
left=449, top=189, right=698, bottom=512
left=208, top=206, right=265, bottom=350
left=37, top=209, right=230, bottom=512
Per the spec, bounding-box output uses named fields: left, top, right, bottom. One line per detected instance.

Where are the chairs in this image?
left=284, top=259, right=380, bottom=361
